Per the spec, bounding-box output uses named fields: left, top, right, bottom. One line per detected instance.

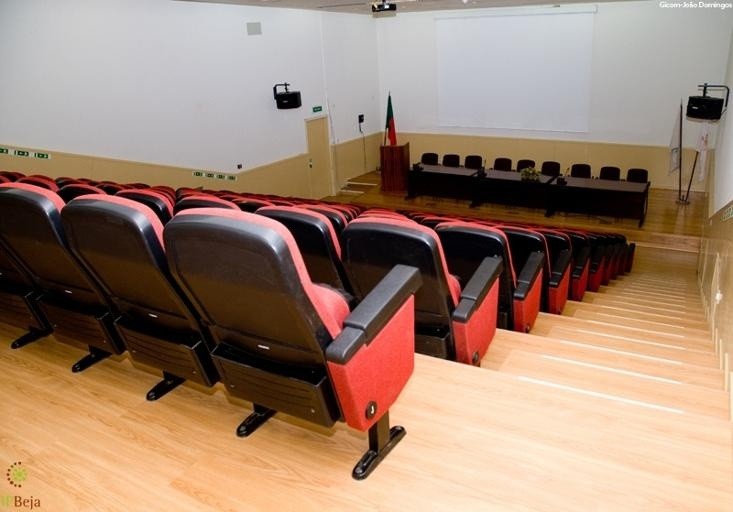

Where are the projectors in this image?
left=371, top=3, right=396, bottom=12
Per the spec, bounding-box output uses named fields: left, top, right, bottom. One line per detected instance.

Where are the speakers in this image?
left=275, top=92, right=301, bottom=110
left=685, top=96, right=724, bottom=120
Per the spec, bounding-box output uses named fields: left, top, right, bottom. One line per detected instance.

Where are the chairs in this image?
left=421, top=152, right=535, bottom=173
left=0, top=169, right=635, bottom=482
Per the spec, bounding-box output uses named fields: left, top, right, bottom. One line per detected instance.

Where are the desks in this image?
left=411, top=162, right=650, bottom=226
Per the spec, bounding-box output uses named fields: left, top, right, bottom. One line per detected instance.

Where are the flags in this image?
left=387, top=96, right=397, bottom=145
left=696, top=121, right=710, bottom=181
left=669, top=109, right=680, bottom=176
